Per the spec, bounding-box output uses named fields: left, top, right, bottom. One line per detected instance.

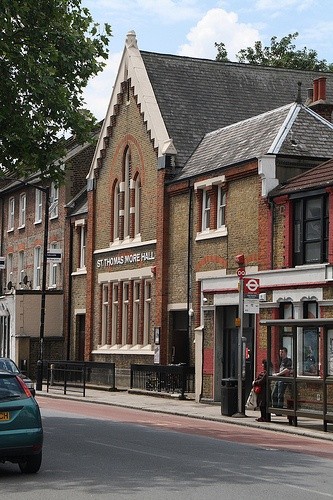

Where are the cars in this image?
left=0, top=367, right=43, bottom=473
left=0, top=357, right=35, bottom=397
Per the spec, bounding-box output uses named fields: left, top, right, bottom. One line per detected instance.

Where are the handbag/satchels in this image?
left=255, top=384, right=262, bottom=394
left=245, top=387, right=258, bottom=411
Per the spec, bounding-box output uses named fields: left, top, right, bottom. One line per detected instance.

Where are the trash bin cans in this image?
left=221, top=377, right=239, bottom=417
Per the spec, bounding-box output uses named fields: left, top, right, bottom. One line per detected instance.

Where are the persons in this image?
left=270, top=347, right=292, bottom=417
left=252, top=359, right=275, bottom=422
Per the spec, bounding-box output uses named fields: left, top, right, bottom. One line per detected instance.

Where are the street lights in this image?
left=0, top=178, right=51, bottom=392
